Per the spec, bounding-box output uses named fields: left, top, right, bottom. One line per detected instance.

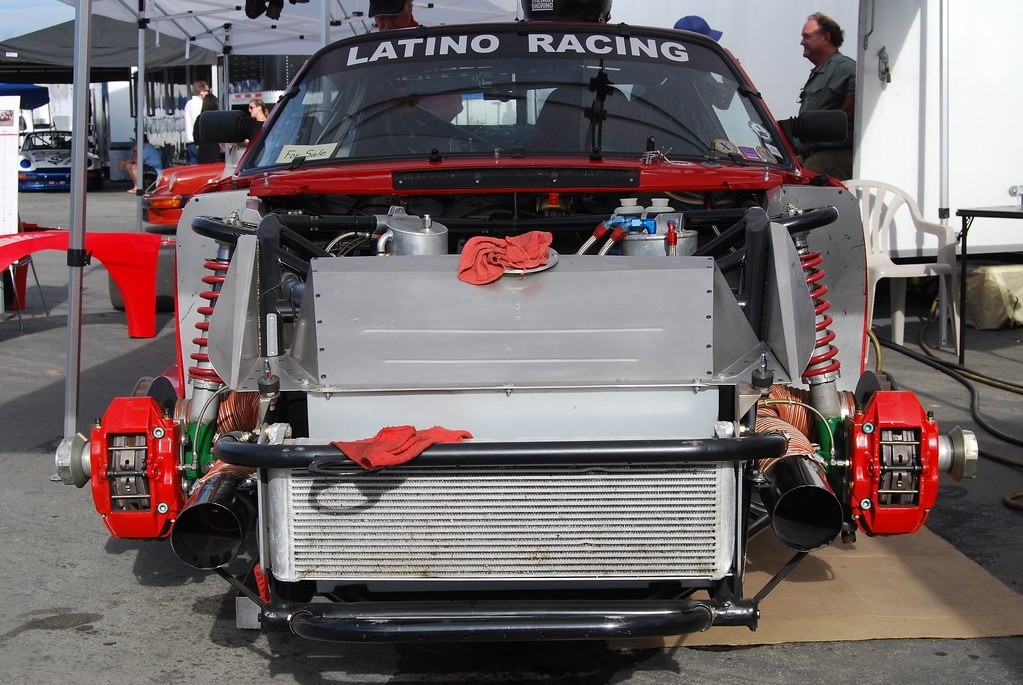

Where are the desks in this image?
left=955, top=203, right=1023, bottom=366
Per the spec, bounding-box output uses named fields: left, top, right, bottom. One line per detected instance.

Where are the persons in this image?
left=116, top=134, right=163, bottom=194
left=193, top=93, right=222, bottom=164
left=630, top=16, right=740, bottom=157
left=367, top=0, right=464, bottom=124
left=183, top=80, right=210, bottom=163
left=792, top=11, right=856, bottom=181
left=249, top=99, right=269, bottom=121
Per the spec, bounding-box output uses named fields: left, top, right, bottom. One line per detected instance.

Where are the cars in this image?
left=17, top=129, right=103, bottom=192
left=53, top=20, right=979, bottom=640
left=136, top=161, right=226, bottom=227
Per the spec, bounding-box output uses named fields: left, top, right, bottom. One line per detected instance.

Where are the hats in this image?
left=673, top=15, right=723, bottom=42
left=368, top=0, right=408, bottom=18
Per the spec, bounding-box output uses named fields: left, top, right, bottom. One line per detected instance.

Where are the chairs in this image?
left=9, top=213, right=51, bottom=331
left=526, top=84, right=647, bottom=149
left=840, top=179, right=959, bottom=357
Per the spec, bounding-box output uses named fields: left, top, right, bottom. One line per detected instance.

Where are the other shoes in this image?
left=128, top=190, right=136, bottom=194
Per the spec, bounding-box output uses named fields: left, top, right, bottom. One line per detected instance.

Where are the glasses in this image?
left=248, top=106, right=257, bottom=110
left=203, top=89, right=210, bottom=94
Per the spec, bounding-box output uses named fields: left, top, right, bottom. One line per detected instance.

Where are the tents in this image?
left=0, top=0, right=524, bottom=480
